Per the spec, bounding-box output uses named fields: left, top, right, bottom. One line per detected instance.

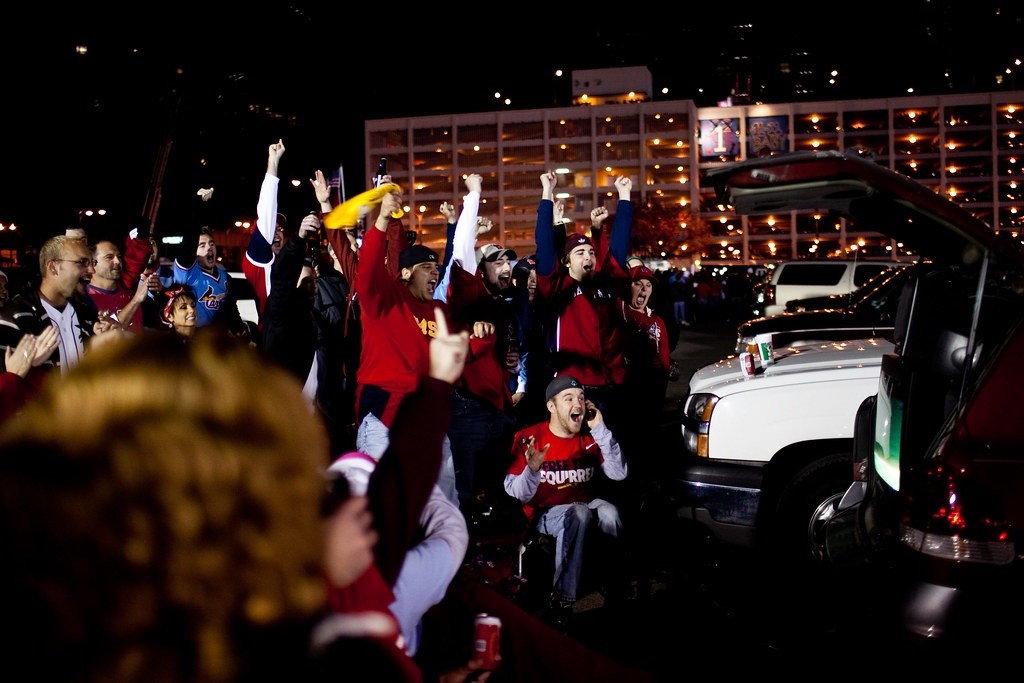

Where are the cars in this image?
left=670, top=337, right=899, bottom=607
left=736, top=264, right=915, bottom=355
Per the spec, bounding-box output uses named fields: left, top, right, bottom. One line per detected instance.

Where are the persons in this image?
left=0, top=235, right=163, bottom=419
left=299, top=157, right=670, bottom=568
left=0, top=305, right=501, bottom=683
left=120, top=139, right=317, bottom=393
left=504, top=375, right=643, bottom=624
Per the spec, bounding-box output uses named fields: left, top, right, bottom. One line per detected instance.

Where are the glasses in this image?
left=45, top=258, right=96, bottom=267
left=275, top=226, right=283, bottom=233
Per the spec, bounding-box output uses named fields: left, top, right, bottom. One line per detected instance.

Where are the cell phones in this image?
left=586, top=409, right=594, bottom=419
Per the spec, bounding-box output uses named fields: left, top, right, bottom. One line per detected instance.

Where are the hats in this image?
left=398, top=245, right=439, bottom=270
left=545, top=376, right=582, bottom=400
left=557, top=232, right=592, bottom=258
left=249, top=213, right=286, bottom=234
left=475, top=243, right=517, bottom=266
left=628, top=266, right=656, bottom=287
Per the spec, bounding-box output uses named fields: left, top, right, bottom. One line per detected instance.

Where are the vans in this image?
left=763, top=261, right=902, bottom=319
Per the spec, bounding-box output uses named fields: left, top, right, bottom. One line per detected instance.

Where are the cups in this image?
left=755, top=335, right=774, bottom=365
left=473, top=618, right=501, bottom=667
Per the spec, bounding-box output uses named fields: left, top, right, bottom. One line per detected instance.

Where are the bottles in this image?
left=374, top=157, right=387, bottom=190
left=306, top=210, right=320, bottom=255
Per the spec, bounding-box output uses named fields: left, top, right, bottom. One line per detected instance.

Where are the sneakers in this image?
left=548, top=591, right=576, bottom=630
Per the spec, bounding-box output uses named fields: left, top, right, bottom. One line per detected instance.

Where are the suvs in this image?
left=699, top=150, right=1024, bottom=683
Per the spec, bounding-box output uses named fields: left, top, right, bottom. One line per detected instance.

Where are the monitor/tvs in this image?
left=872, top=354, right=914, bottom=493
